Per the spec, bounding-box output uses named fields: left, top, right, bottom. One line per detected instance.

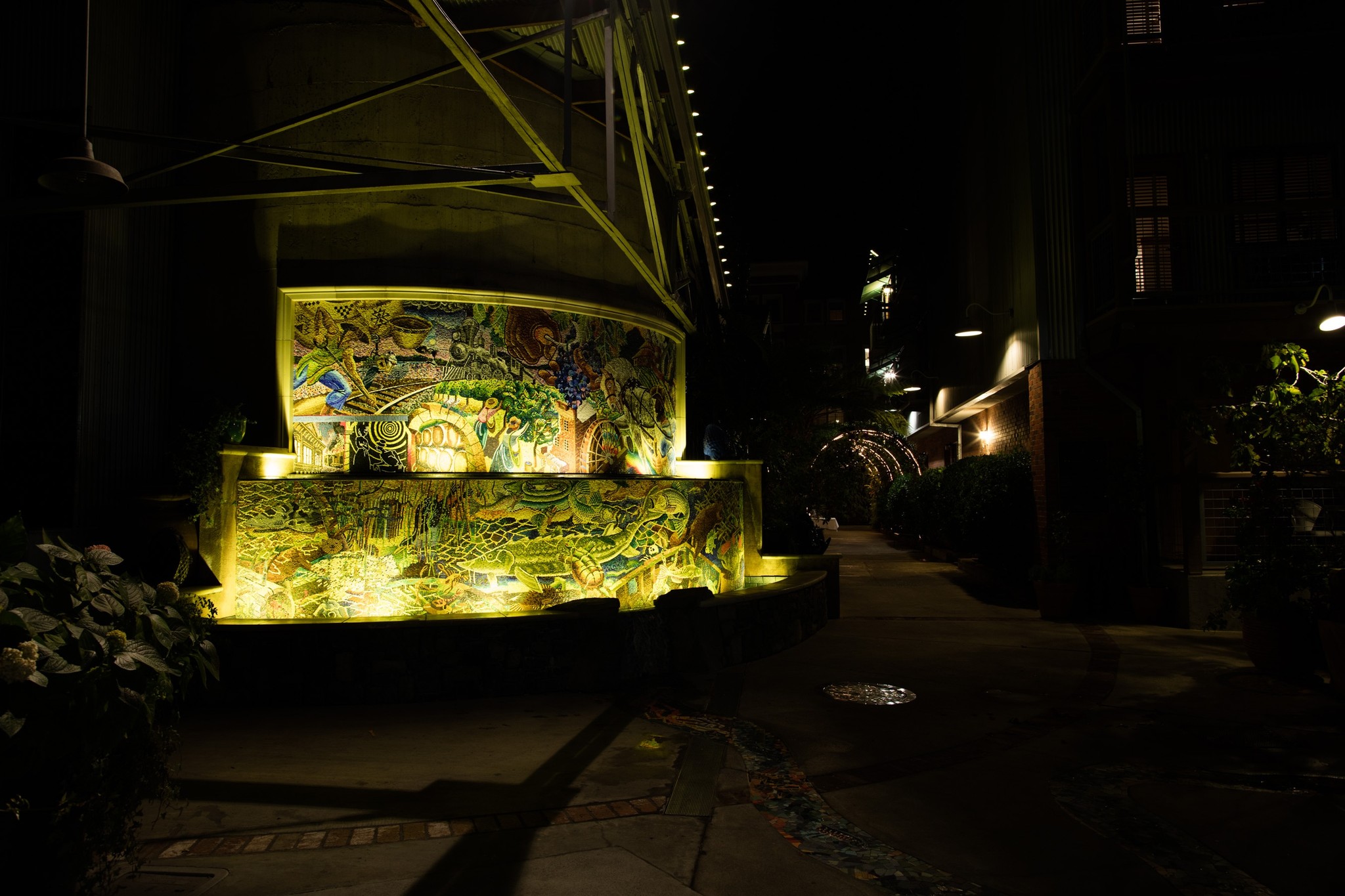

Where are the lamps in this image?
left=37, top=0, right=130, bottom=198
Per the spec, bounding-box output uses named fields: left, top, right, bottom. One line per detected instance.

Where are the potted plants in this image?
left=1185, top=341, right=1345, bottom=695
left=1030, top=506, right=1087, bottom=621
left=1100, top=444, right=1170, bottom=625
